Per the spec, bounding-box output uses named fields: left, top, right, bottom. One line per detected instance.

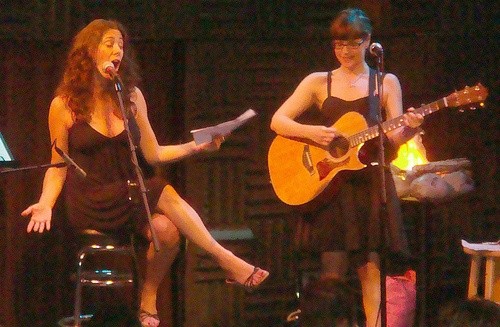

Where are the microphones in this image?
left=54, top=145, right=86, bottom=180
left=101, top=60, right=124, bottom=88
left=369, top=41, right=384, bottom=59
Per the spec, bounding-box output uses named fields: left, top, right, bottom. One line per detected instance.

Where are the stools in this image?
left=461, top=240, right=500, bottom=307
left=69, top=229, right=139, bottom=327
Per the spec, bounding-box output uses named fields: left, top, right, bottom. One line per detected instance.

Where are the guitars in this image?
left=268, top=82, right=489, bottom=214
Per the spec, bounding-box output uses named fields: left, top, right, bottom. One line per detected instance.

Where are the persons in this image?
left=270, top=7, right=424, bottom=327
left=20, top=16, right=271, bottom=327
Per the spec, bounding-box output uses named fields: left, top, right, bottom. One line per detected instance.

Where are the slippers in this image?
left=224, top=266, right=269, bottom=288
left=137, top=298, right=160, bottom=326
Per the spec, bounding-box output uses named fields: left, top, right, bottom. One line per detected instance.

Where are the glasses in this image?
left=331, top=34, right=370, bottom=50
